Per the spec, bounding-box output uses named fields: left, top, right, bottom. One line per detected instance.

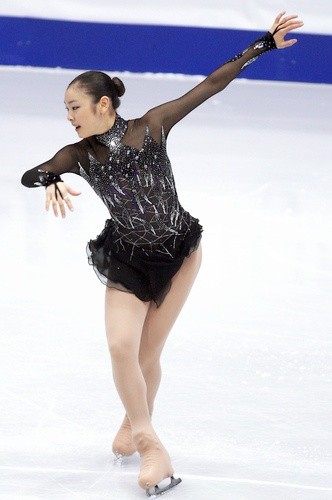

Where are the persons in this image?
left=21, top=11, right=305, bottom=496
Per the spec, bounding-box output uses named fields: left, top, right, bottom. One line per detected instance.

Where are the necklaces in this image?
left=94, top=114, right=128, bottom=150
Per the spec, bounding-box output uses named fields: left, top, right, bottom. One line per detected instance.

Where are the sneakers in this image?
left=131, top=428, right=182, bottom=500
left=111, top=403, right=155, bottom=466
